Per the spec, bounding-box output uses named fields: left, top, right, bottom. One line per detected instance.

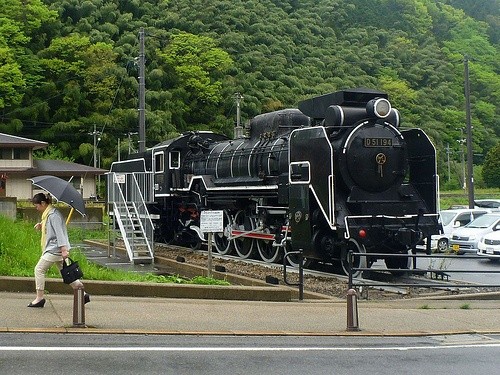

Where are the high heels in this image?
left=84, top=293, right=90, bottom=304
left=28, top=298, right=46, bottom=307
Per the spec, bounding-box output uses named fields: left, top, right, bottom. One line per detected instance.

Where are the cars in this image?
left=448, top=213, right=500, bottom=255
left=447, top=204, right=481, bottom=210
left=476, top=230, right=500, bottom=262
left=473, top=199, right=500, bottom=209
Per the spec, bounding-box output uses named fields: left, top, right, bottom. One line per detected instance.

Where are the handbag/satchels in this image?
left=60, top=257, right=83, bottom=284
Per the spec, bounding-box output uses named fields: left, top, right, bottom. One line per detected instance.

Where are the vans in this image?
left=415, top=209, right=500, bottom=254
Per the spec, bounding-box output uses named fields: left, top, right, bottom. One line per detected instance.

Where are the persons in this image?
left=27, top=193, right=90, bottom=307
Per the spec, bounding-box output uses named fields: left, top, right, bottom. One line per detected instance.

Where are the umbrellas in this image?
left=26, top=175, right=86, bottom=228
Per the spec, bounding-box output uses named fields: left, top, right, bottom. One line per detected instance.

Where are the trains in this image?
left=124, top=87, right=445, bottom=280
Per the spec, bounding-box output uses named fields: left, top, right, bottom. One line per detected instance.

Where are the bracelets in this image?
left=61, top=249, right=66, bottom=251
